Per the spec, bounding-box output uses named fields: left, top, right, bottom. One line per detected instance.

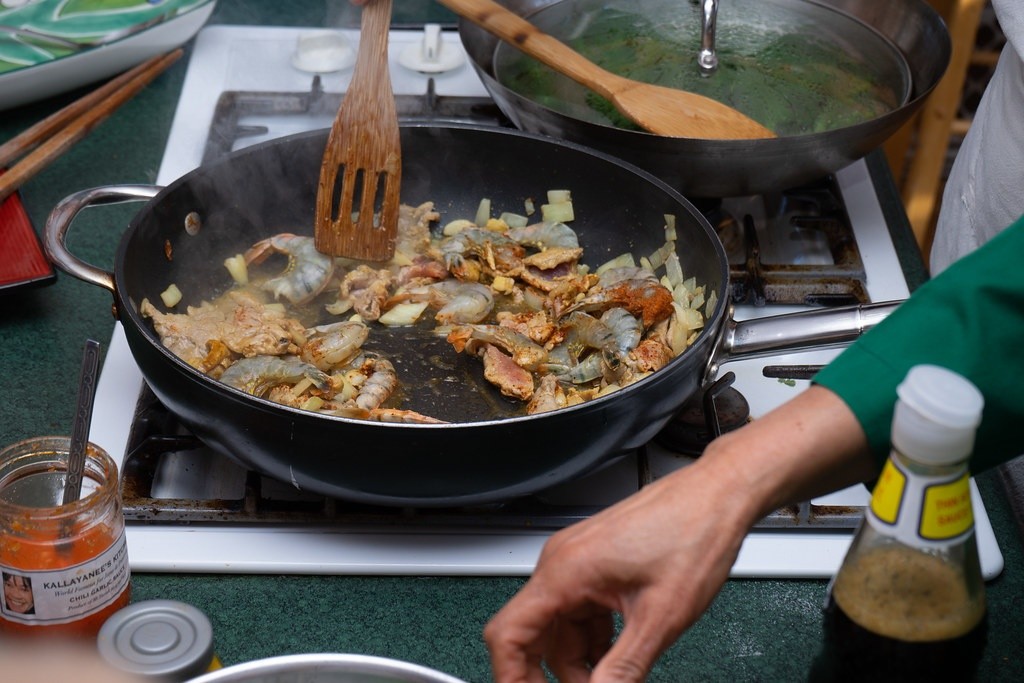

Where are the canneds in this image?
left=1, top=437, right=128, bottom=643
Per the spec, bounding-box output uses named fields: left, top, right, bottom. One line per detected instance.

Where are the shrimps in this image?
left=220, top=222, right=673, bottom=427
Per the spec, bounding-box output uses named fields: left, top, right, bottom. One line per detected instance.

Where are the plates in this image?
left=0, top=164, right=58, bottom=301
left=1, top=0, right=217, bottom=109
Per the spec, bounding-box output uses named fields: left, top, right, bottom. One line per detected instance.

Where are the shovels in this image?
left=437, top=0, right=779, bottom=142
left=314, top=1, right=403, bottom=265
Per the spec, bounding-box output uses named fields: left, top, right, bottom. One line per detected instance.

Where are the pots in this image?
left=457, top=1, right=953, bottom=198
left=42, top=110, right=904, bottom=511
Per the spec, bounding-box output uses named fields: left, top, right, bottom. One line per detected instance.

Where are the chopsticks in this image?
left=0, top=49, right=187, bottom=207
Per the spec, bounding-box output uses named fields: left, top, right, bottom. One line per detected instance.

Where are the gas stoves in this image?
left=64, top=23, right=919, bottom=581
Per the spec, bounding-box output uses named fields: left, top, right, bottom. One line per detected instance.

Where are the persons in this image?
left=480, top=208, right=1020, bottom=683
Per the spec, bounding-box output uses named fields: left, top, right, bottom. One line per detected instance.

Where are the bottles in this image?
left=817, top=363, right=992, bottom=683
left=0, top=437, right=133, bottom=648
left=95, top=598, right=221, bottom=683
